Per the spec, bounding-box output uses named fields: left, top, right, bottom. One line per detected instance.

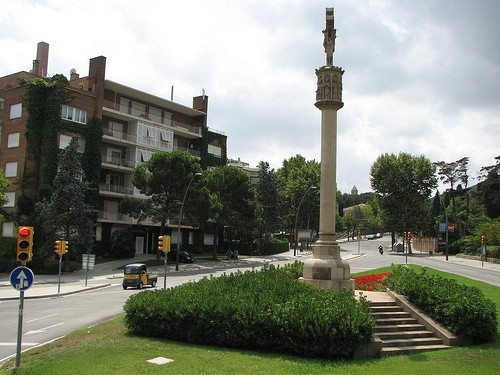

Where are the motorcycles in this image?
left=379, top=247, right=383, bottom=255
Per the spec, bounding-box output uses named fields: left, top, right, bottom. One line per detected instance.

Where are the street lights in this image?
left=412, top=181, right=448, bottom=261
left=176, top=173, right=203, bottom=271
left=294, top=186, right=317, bottom=255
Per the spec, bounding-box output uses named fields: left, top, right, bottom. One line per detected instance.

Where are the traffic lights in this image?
left=17, top=226, right=32, bottom=261
left=403, top=232, right=406, bottom=240
left=54, top=241, right=61, bottom=255
left=158, top=235, right=167, bottom=253
left=481, top=236, right=484, bottom=244
left=61, top=241, right=69, bottom=254
left=408, top=232, right=411, bottom=240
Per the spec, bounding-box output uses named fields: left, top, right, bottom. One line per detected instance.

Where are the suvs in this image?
left=168, top=251, right=197, bottom=264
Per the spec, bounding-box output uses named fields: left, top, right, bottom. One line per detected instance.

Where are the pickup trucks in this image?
left=122, top=264, right=158, bottom=290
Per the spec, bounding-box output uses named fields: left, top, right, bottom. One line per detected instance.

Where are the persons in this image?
left=233, top=248, right=238, bottom=263
left=379, top=246, right=382, bottom=250
left=226, top=248, right=233, bottom=262
